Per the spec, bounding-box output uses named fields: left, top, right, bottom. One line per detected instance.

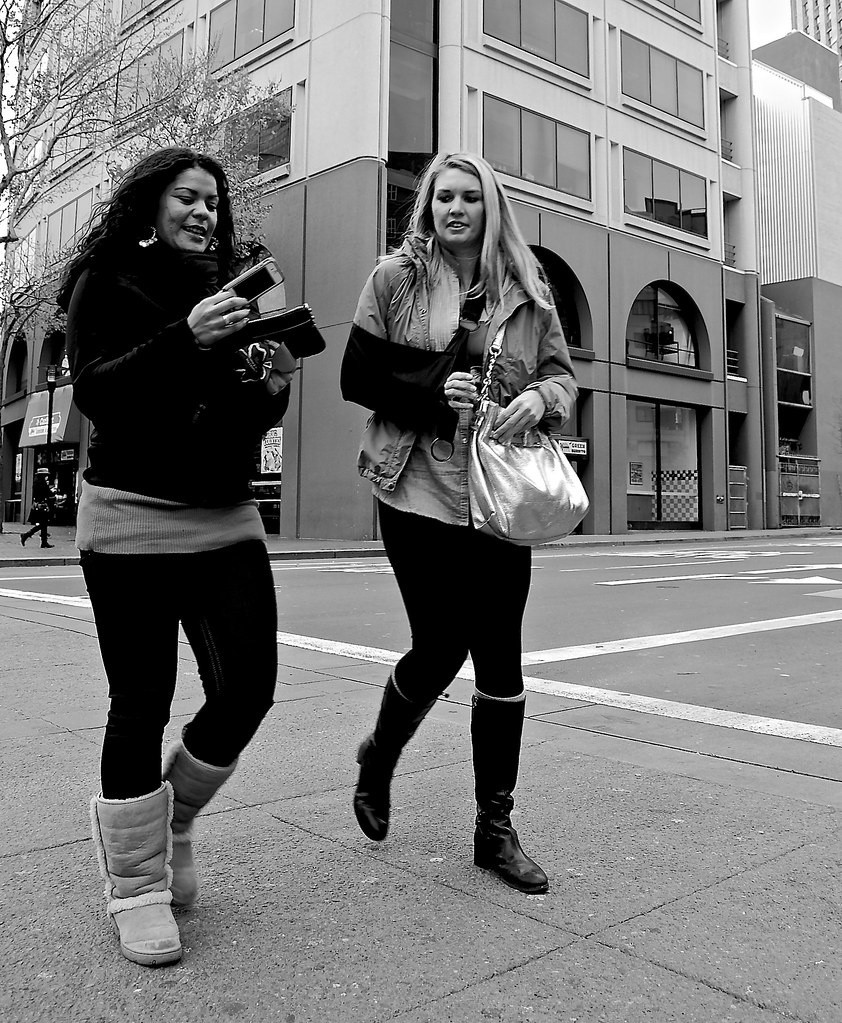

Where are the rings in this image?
left=223, top=315, right=229, bottom=325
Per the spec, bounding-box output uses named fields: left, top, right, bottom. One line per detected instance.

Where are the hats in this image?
left=35, top=468, right=50, bottom=474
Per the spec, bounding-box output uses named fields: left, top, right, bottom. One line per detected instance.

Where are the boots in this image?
left=163, top=720, right=240, bottom=906
left=355, top=665, right=438, bottom=842
left=471, top=694, right=549, bottom=893
left=40, top=534, right=55, bottom=548
left=91, top=779, right=183, bottom=965
left=20, top=529, right=35, bottom=546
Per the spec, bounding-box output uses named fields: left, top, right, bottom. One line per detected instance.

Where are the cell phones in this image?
left=222, top=257, right=285, bottom=310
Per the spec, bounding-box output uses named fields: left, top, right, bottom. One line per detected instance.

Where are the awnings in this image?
left=19, top=385, right=79, bottom=448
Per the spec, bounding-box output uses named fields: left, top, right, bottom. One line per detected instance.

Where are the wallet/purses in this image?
left=218, top=303, right=325, bottom=373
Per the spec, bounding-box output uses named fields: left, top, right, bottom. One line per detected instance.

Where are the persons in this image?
left=340, top=149, right=578, bottom=896
left=58, top=148, right=291, bottom=965
left=20, top=468, right=64, bottom=548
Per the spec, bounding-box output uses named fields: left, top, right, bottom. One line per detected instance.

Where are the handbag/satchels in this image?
left=466, top=396, right=590, bottom=547
